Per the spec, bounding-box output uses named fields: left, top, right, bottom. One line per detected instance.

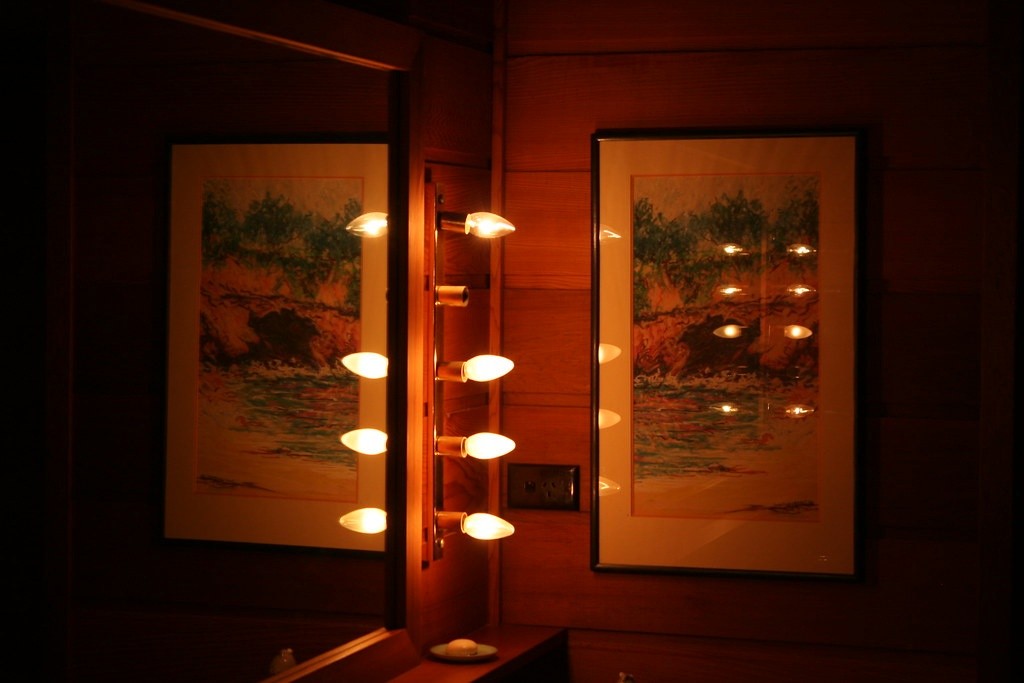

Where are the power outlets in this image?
left=507, top=462, right=580, bottom=512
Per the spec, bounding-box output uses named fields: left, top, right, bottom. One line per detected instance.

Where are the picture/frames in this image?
left=155, top=132, right=390, bottom=562
left=589, top=128, right=866, bottom=583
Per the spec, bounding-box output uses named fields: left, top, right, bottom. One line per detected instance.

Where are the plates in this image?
left=430, top=644, right=498, bottom=662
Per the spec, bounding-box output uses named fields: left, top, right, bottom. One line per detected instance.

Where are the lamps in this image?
left=776, top=243, right=816, bottom=258
left=772, top=282, right=816, bottom=301
left=715, top=243, right=762, bottom=259
left=341, top=352, right=389, bottom=380
left=713, top=324, right=758, bottom=338
left=772, top=325, right=813, bottom=340
left=337, top=427, right=388, bottom=456
left=435, top=432, right=517, bottom=460
left=773, top=401, right=814, bottom=419
left=345, top=212, right=388, bottom=239
left=437, top=511, right=515, bottom=541
left=438, top=354, right=514, bottom=383
left=442, top=211, right=516, bottom=238
left=339, top=507, right=387, bottom=535
left=716, top=285, right=762, bottom=305
left=709, top=402, right=758, bottom=419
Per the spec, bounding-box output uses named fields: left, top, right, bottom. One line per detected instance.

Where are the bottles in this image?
left=269, top=646, right=296, bottom=677
left=616, top=671, right=636, bottom=683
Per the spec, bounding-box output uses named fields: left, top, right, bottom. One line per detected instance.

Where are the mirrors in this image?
left=0, top=0, right=408, bottom=683
